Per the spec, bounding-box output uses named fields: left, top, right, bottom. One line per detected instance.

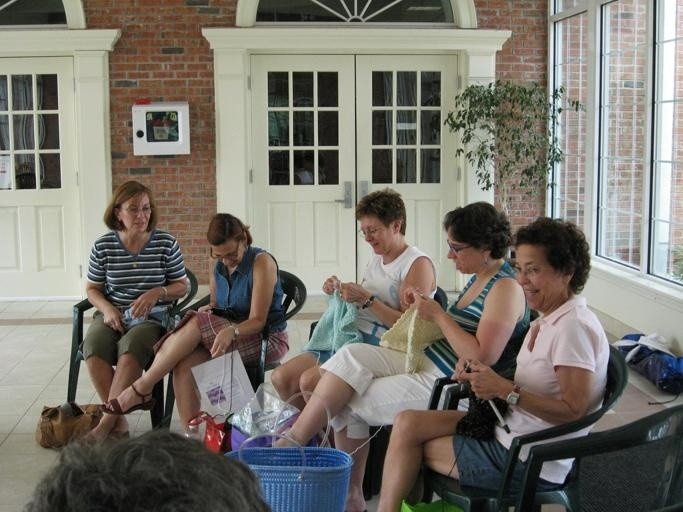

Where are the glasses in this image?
left=118, top=204, right=150, bottom=213
left=446, top=238, right=472, bottom=253
left=209, top=241, right=240, bottom=259
left=359, top=225, right=387, bottom=236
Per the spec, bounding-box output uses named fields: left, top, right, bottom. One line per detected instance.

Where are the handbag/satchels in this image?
left=232, top=408, right=318, bottom=449
left=35, top=400, right=129, bottom=448
left=188, top=411, right=232, bottom=453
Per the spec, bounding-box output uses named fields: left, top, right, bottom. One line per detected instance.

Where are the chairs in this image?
left=69, top=266, right=198, bottom=429
left=393, top=299, right=539, bottom=415
left=519, top=406, right=683, bottom=511
left=418, top=339, right=626, bottom=511
left=310, top=287, right=449, bottom=379
left=174, top=268, right=306, bottom=431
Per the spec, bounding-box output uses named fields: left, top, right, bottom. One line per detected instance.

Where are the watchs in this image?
left=362, top=296, right=376, bottom=309
left=506, top=385, right=520, bottom=406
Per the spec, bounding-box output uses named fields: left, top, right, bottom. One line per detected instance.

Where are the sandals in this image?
left=100, top=385, right=155, bottom=416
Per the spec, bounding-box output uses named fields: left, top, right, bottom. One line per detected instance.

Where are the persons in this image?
left=273, top=200, right=533, bottom=511
left=375, top=216, right=611, bottom=511
left=271, top=185, right=439, bottom=446
left=24, top=428, right=273, bottom=511
left=99, top=212, right=290, bottom=442
left=81, top=179, right=188, bottom=446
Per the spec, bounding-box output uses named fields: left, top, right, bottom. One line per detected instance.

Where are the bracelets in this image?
left=229, top=325, right=239, bottom=343
left=159, top=286, right=168, bottom=303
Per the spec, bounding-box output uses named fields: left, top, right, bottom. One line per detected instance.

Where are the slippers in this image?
left=80, top=427, right=128, bottom=447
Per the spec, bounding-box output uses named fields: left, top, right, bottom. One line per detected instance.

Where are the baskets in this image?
left=239, top=392, right=354, bottom=512
